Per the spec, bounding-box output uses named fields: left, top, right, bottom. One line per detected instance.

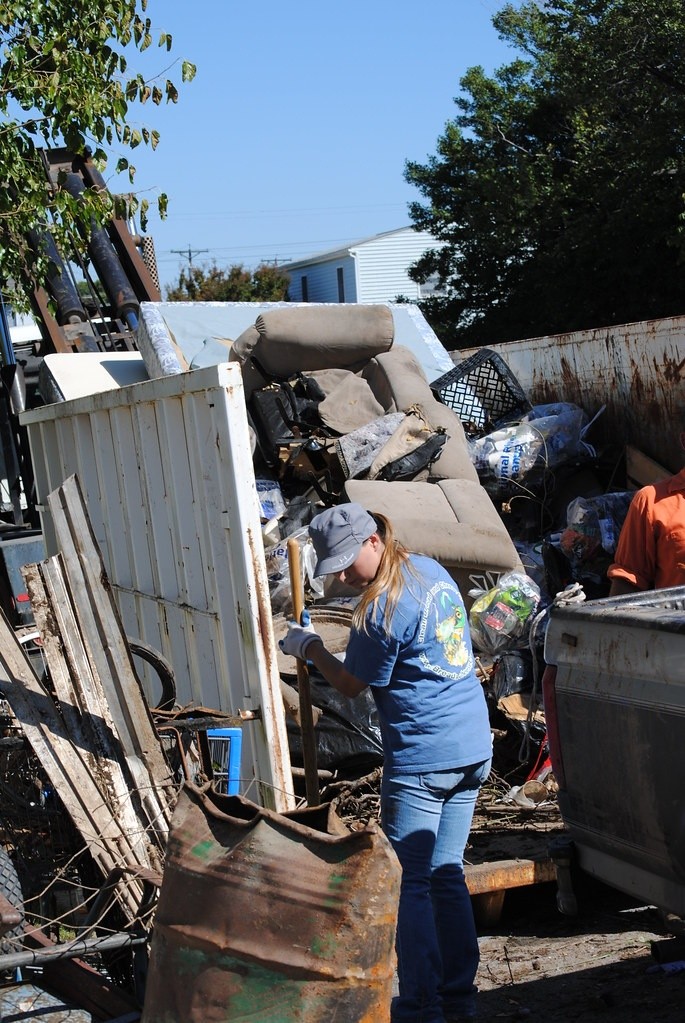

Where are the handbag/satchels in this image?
left=468, top=569, right=541, bottom=655
left=364, top=403, right=452, bottom=482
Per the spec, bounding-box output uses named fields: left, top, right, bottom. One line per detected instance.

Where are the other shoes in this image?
left=441, top=1006, right=476, bottom=1020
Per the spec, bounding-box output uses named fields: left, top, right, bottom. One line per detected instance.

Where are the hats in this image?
left=308, top=502, right=378, bottom=579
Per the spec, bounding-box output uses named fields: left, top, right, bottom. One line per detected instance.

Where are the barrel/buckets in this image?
left=516, top=780, right=548, bottom=804
left=140, top=782, right=401, bottom=1023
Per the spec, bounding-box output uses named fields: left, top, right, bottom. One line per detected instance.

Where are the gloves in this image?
left=278, top=620, right=323, bottom=661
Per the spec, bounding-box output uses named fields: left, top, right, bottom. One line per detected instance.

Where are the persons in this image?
left=607, top=430, right=684, bottom=596
left=278, top=503, right=493, bottom=1023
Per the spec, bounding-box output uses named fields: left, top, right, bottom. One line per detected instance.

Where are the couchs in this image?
left=229, top=302, right=527, bottom=616
